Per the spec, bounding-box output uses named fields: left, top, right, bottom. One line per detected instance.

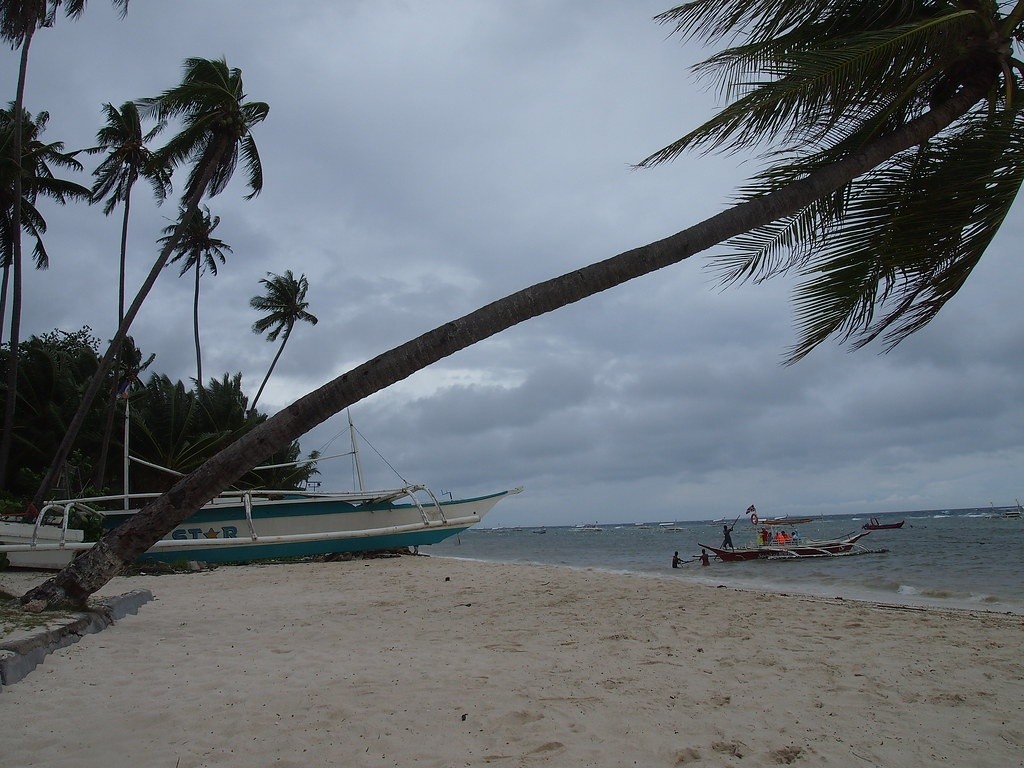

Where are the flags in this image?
left=746, top=505, right=755, bottom=515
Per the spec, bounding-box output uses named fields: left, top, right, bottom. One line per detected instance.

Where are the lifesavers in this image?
left=751, top=514, right=758, bottom=525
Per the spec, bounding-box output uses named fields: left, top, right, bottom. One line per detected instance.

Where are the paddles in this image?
left=714, top=514, right=741, bottom=560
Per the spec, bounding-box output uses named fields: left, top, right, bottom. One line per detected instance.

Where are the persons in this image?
left=673, top=551, right=679, bottom=568
left=699, top=549, right=710, bottom=567
left=757, top=527, right=799, bottom=545
left=22, top=503, right=39, bottom=524
left=723, top=526, right=734, bottom=552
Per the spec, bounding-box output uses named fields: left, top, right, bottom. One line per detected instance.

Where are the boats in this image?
left=700, top=531, right=868, bottom=560
left=759, top=516, right=813, bottom=525
left=0, top=392, right=526, bottom=568
left=530, top=526, right=548, bottom=533
left=862, top=516, right=904, bottom=531
left=570, top=522, right=603, bottom=531
